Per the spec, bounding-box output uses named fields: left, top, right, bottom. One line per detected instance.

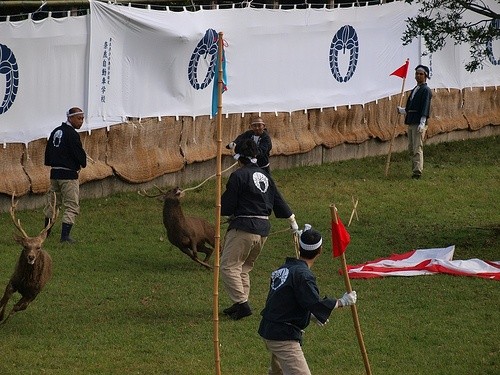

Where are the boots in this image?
left=61, top=222, right=75, bottom=242
left=231, top=301, right=252, bottom=319
left=44, top=216, right=55, bottom=237
left=223, top=303, right=239, bottom=314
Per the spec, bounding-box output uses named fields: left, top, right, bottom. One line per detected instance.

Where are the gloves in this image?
left=417, top=116, right=426, bottom=134
left=397, top=106, right=406, bottom=114
left=287, top=213, right=298, bottom=231
left=338, top=290, right=357, bottom=307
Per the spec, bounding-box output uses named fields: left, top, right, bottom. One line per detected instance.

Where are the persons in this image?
left=43, top=107, right=86, bottom=242
left=220, top=118, right=299, bottom=320
left=258, top=229, right=356, bottom=375
left=397, top=65, right=432, bottom=179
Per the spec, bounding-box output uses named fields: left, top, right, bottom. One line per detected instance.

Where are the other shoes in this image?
left=412, top=170, right=421, bottom=177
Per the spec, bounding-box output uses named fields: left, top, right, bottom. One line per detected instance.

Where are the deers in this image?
left=0, top=190, right=60, bottom=327
left=131, top=171, right=223, bottom=272
